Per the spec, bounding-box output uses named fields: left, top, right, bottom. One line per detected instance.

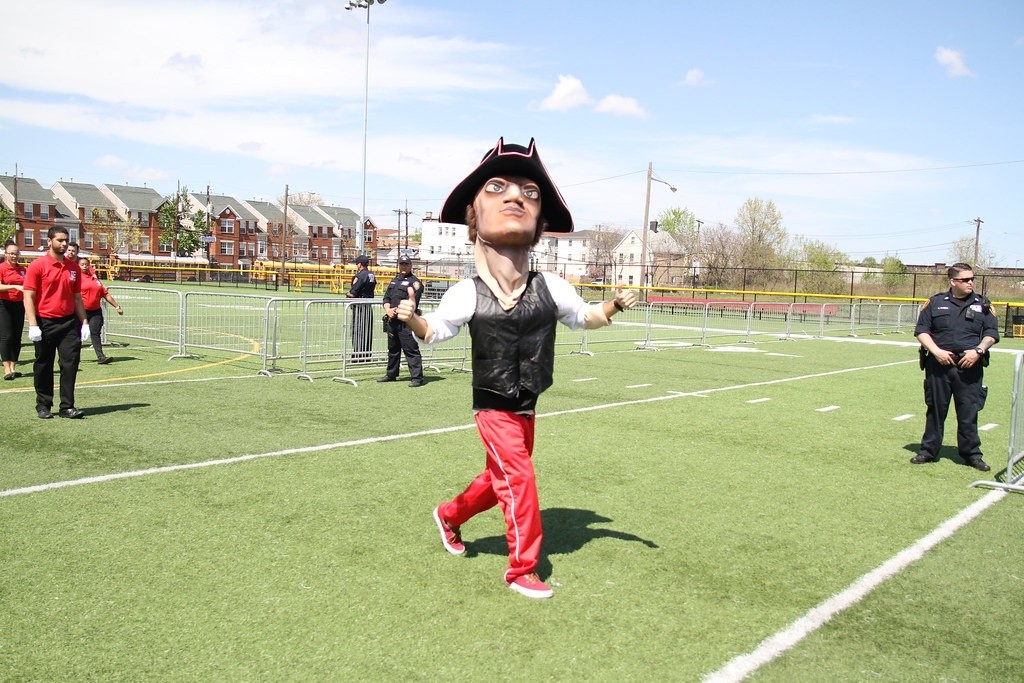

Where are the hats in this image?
left=357, top=255, right=369, bottom=262
left=399, top=255, right=411, bottom=263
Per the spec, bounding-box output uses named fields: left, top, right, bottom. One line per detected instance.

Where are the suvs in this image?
left=378, top=244, right=421, bottom=270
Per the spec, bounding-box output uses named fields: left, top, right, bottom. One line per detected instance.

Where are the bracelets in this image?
left=116, top=306, right=121, bottom=310
left=614, top=300, right=624, bottom=312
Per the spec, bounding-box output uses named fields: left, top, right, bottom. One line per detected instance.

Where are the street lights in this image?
left=638, top=178, right=678, bottom=302
left=344, top=0, right=387, bottom=248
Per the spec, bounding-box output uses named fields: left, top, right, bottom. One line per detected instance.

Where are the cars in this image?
left=591, top=277, right=612, bottom=291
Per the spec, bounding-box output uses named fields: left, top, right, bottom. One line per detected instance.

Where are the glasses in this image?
left=954, top=277, right=975, bottom=282
left=8, top=252, right=19, bottom=257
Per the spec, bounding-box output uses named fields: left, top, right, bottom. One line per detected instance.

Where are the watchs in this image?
left=975, top=347, right=983, bottom=357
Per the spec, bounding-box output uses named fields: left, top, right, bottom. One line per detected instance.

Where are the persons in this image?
left=910, top=263, right=1000, bottom=471
left=396, top=138, right=636, bottom=599
left=346, top=255, right=377, bottom=363
left=23, top=226, right=90, bottom=418
left=0, top=239, right=26, bottom=380
left=66, top=242, right=95, bottom=277
left=78, top=258, right=123, bottom=364
left=377, top=255, right=424, bottom=388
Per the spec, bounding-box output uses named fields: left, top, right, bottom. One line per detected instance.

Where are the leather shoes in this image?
left=967, top=459, right=990, bottom=470
left=911, top=454, right=939, bottom=464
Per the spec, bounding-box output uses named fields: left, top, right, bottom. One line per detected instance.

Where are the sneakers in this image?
left=510, top=572, right=553, bottom=598
left=433, top=506, right=465, bottom=555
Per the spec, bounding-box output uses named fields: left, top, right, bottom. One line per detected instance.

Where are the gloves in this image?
left=29, top=326, right=42, bottom=342
left=81, top=324, right=90, bottom=341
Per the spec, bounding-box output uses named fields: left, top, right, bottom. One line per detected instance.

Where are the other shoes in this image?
left=59, top=407, right=84, bottom=418
left=4, top=374, right=12, bottom=380
left=377, top=375, right=396, bottom=381
left=38, top=410, right=54, bottom=418
left=408, top=380, right=423, bottom=387
left=12, top=372, right=22, bottom=377
left=98, top=357, right=113, bottom=364
